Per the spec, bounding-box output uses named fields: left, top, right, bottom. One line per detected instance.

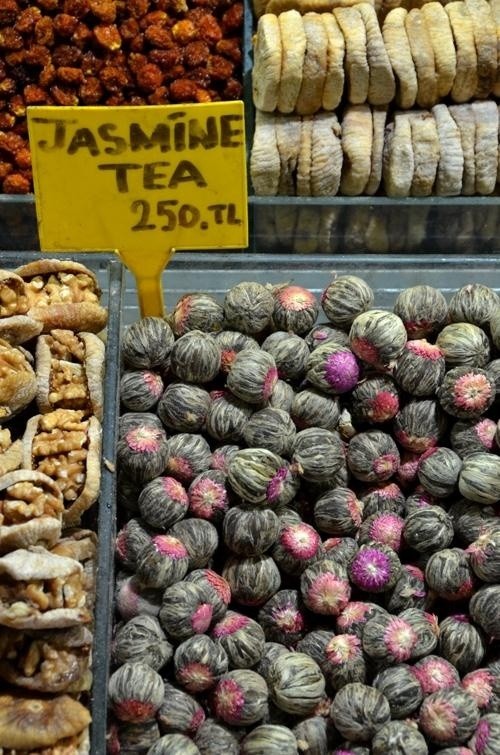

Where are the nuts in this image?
left=0, top=257, right=107, bottom=754
